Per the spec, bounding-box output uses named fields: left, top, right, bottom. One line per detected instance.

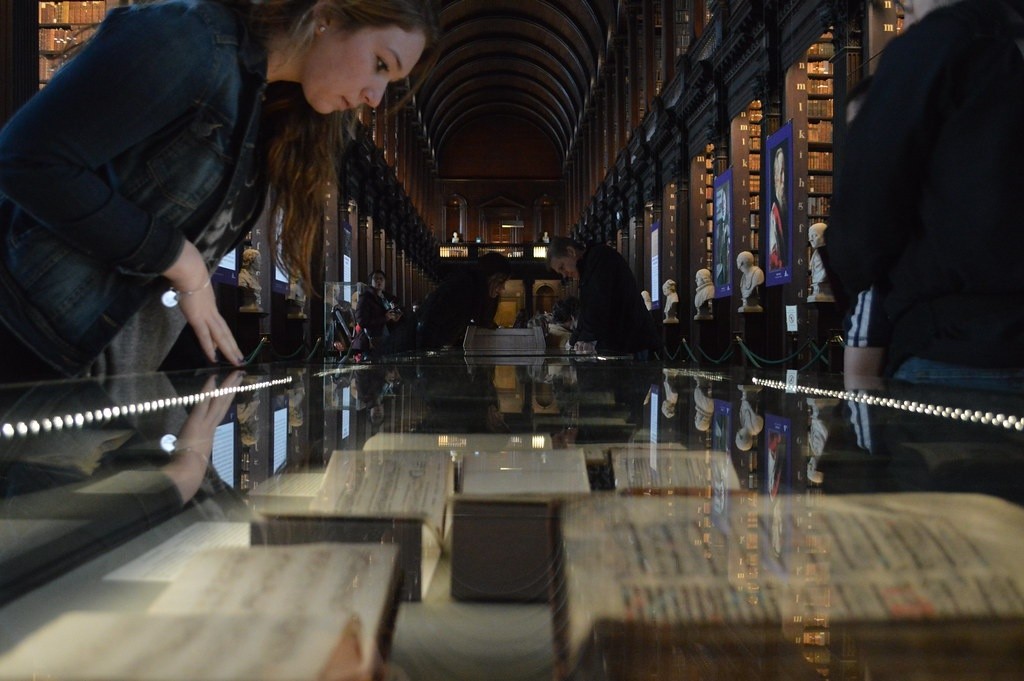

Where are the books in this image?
left=38, top=0, right=119, bottom=92
left=0, top=445, right=1024, bottom=681
left=650, top=0, right=906, bottom=287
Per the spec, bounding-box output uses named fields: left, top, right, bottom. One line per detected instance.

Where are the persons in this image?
left=1, top=1, right=435, bottom=384
left=715, top=186, right=731, bottom=285
left=326, top=283, right=340, bottom=306
left=288, top=397, right=303, bottom=427
left=356, top=269, right=404, bottom=358
left=452, top=231, right=459, bottom=244
left=695, top=269, right=714, bottom=313
left=842, top=376, right=1023, bottom=458
left=768, top=148, right=788, bottom=270
left=808, top=223, right=827, bottom=296
left=737, top=251, right=764, bottom=302
left=2, top=370, right=243, bottom=590
left=238, top=248, right=261, bottom=307
left=419, top=250, right=509, bottom=350
left=547, top=237, right=658, bottom=353
left=662, top=279, right=678, bottom=314
left=237, top=399, right=260, bottom=446
left=543, top=231, right=549, bottom=243
left=641, top=291, right=651, bottom=311
left=817, top=1, right=1024, bottom=376
left=351, top=290, right=357, bottom=310
left=355, top=358, right=656, bottom=464
left=351, top=378, right=358, bottom=399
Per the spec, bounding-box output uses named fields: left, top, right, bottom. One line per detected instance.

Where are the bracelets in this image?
left=176, top=448, right=209, bottom=467
left=174, top=275, right=211, bottom=302
